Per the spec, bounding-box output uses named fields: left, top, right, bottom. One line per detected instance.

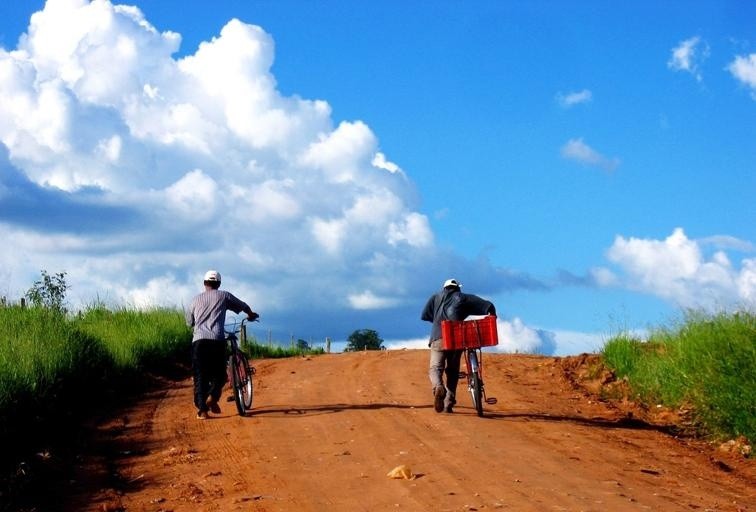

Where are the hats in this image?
left=443, top=279, right=462, bottom=288
left=204, top=270, right=222, bottom=281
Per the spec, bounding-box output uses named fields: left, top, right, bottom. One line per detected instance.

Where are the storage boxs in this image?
left=441, top=315, right=499, bottom=351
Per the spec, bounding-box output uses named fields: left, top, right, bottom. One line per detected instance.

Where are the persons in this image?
left=185, top=269, right=260, bottom=420
left=421, top=278, right=497, bottom=413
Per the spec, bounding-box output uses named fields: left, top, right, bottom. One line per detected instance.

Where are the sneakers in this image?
left=433, top=386, right=456, bottom=413
left=206, top=396, right=221, bottom=414
left=196, top=411, right=209, bottom=419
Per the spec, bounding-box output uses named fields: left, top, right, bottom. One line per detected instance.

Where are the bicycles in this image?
left=455, top=347, right=497, bottom=416
left=221, top=316, right=259, bottom=417
